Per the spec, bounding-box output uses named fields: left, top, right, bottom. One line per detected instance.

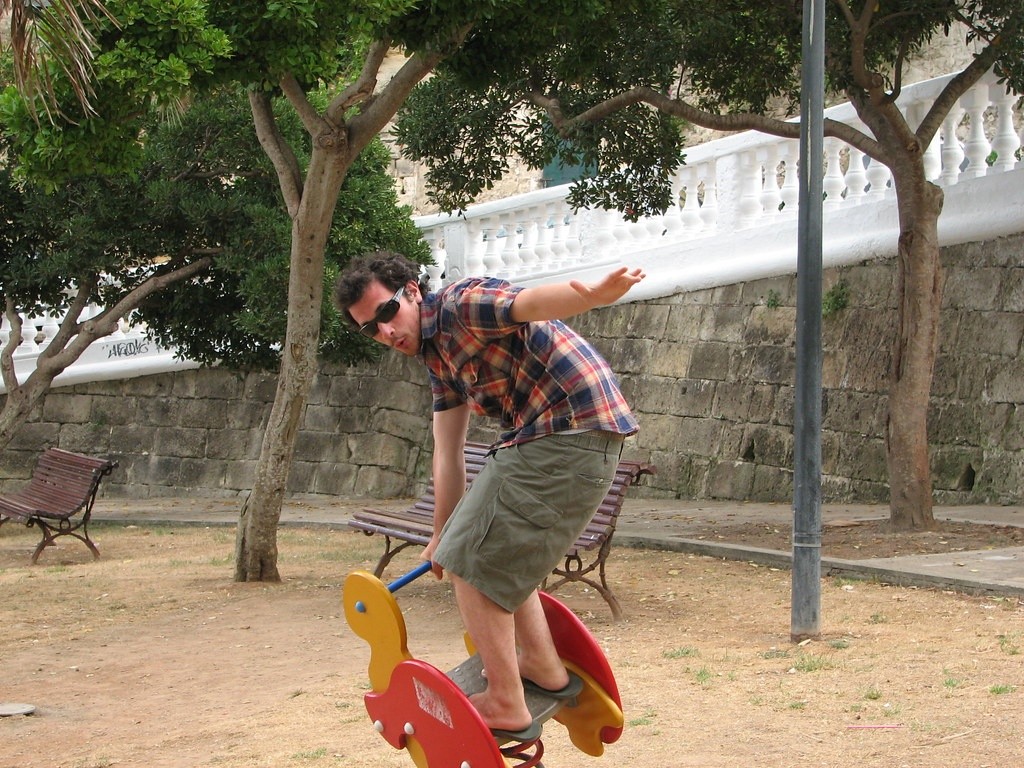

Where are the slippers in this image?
left=466, top=692, right=543, bottom=742
left=522, top=671, right=585, bottom=699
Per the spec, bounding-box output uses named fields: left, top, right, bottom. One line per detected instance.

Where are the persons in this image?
left=330, top=250, right=648, bottom=735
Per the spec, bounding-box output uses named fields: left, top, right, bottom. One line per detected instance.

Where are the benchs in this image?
left=0, top=447, right=119, bottom=564
left=348, top=443, right=655, bottom=620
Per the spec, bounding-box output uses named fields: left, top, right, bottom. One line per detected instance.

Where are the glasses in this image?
left=359, top=286, right=406, bottom=340
